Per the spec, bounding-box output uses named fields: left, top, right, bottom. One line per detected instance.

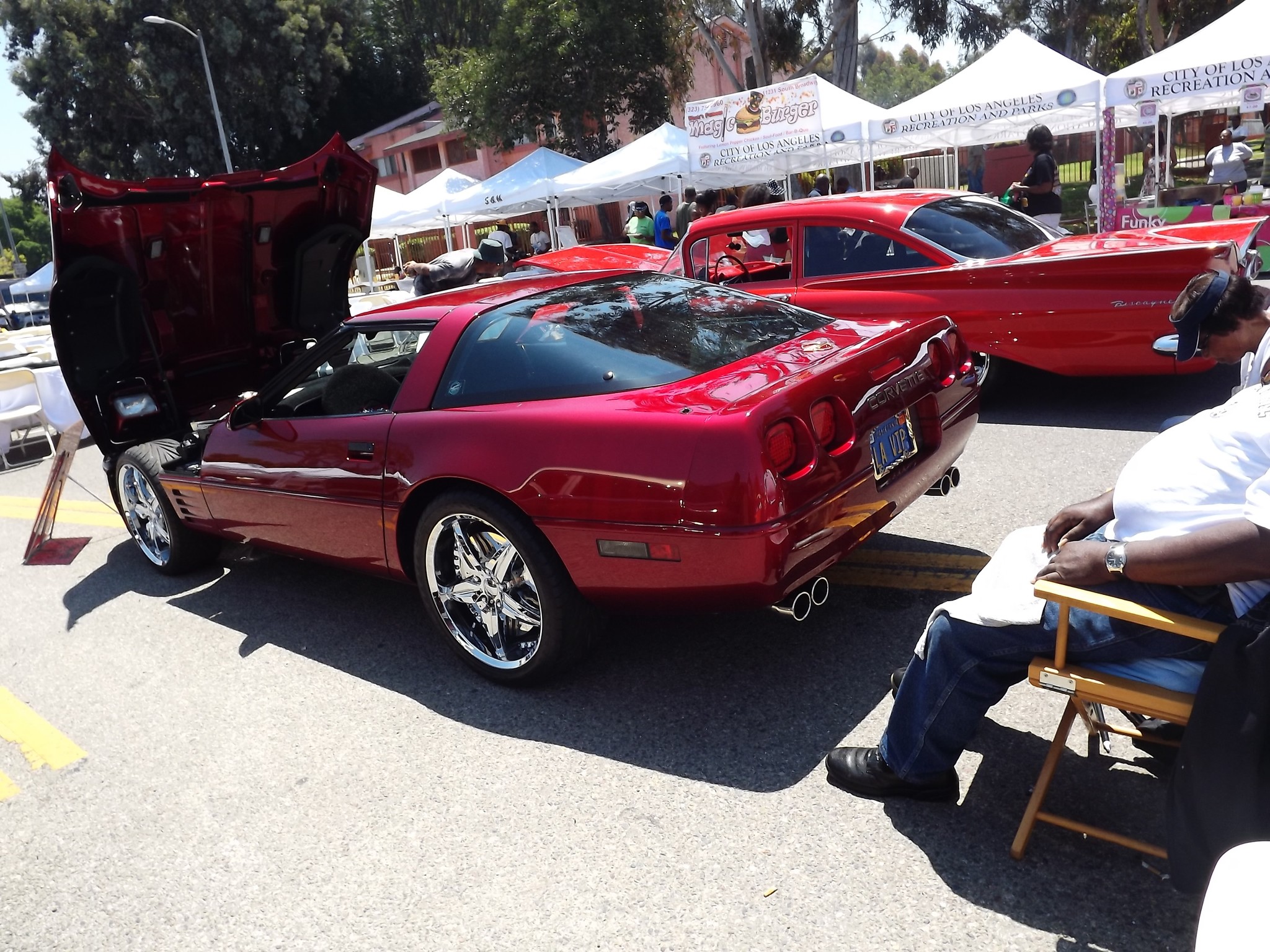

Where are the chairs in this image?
left=0, top=365, right=57, bottom=472
left=1158, top=184, right=1222, bottom=210
left=850, top=236, right=890, bottom=272
left=1011, top=576, right=1225, bottom=860
left=804, top=238, right=849, bottom=280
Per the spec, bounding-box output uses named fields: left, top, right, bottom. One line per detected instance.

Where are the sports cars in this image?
left=507, top=187, right=1270, bottom=408
left=41, top=144, right=985, bottom=688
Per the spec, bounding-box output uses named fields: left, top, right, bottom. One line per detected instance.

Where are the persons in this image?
left=828, top=375, right=1270, bottom=802
left=872, top=163, right=888, bottom=191
left=1227, top=115, right=1247, bottom=144
left=1140, top=130, right=1178, bottom=197
left=488, top=220, right=520, bottom=260
left=835, top=176, right=863, bottom=244
left=1214, top=186, right=1236, bottom=205
left=897, top=165, right=922, bottom=189
left=1011, top=124, right=1062, bottom=228
left=1088, top=139, right=1104, bottom=207
left=619, top=177, right=789, bottom=250
left=1205, top=130, right=1254, bottom=194
left=1156, top=269, right=1269, bottom=435
left=402, top=237, right=505, bottom=298
left=807, top=173, right=842, bottom=262
left=528, top=222, right=550, bottom=255
left=505, top=223, right=521, bottom=253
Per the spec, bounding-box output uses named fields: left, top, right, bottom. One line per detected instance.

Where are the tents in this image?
left=446, top=146, right=591, bottom=250
left=551, top=120, right=689, bottom=252
left=684, top=68, right=887, bottom=200
left=368, top=166, right=482, bottom=270
left=1102, top=0, right=1268, bottom=222
left=355, top=182, right=404, bottom=294
left=863, top=29, right=1110, bottom=224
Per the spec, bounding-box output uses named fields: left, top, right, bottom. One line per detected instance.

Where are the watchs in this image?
left=1103, top=541, right=1129, bottom=582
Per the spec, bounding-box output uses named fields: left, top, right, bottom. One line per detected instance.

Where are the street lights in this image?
left=143, top=15, right=234, bottom=173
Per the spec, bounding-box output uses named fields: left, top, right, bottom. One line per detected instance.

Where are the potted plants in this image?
left=354, top=247, right=376, bottom=283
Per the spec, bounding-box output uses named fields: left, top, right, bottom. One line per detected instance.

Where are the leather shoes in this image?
left=889, top=668, right=909, bottom=690
left=826, top=747, right=959, bottom=795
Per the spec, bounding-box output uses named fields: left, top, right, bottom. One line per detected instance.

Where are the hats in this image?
left=473, top=238, right=508, bottom=263
left=1169, top=269, right=1233, bottom=362
left=496, top=219, right=508, bottom=225
left=632, top=201, right=647, bottom=211
left=767, top=178, right=786, bottom=196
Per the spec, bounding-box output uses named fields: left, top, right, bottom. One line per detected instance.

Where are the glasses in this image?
left=1197, top=334, right=1210, bottom=357
